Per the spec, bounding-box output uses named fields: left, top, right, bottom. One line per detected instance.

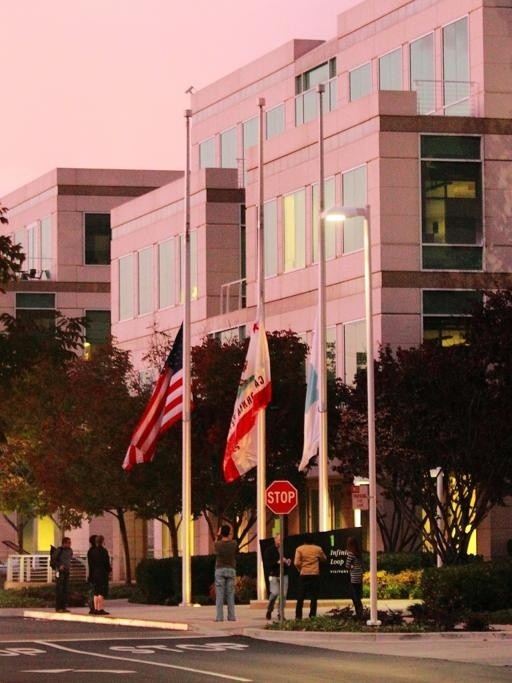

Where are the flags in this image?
left=222, top=309, right=274, bottom=485
left=121, top=322, right=195, bottom=471
left=294, top=313, right=330, bottom=473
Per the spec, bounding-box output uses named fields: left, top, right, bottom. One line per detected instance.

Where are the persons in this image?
left=344, top=535, right=365, bottom=617
left=212, top=523, right=239, bottom=622
left=265, top=532, right=291, bottom=621
left=87, top=535, right=100, bottom=614
left=92, top=535, right=113, bottom=615
left=293, top=533, right=327, bottom=620
left=50, top=536, right=73, bottom=613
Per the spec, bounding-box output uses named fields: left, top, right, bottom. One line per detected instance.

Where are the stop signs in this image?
left=265, top=479, right=298, bottom=515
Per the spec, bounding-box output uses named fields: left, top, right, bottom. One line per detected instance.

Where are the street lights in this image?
left=323, top=204, right=382, bottom=628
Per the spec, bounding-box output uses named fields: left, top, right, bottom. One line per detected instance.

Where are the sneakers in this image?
left=89, top=606, right=109, bottom=614
left=278, top=614, right=285, bottom=620
left=267, top=611, right=271, bottom=619
left=57, top=607, right=70, bottom=613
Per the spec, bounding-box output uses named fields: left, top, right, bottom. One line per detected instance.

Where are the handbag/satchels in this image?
left=50, top=545, right=63, bottom=570
